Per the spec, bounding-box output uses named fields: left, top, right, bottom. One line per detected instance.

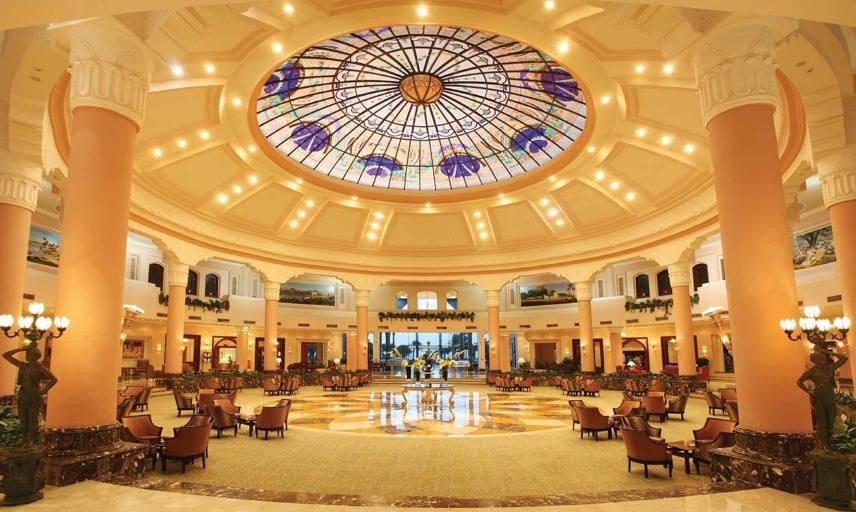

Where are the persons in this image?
left=796, top=345, right=848, bottom=456
left=2, top=342, right=58, bottom=448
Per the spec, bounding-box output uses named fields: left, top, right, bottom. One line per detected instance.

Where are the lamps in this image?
left=780, top=303, right=854, bottom=343
left=0, top=298, right=74, bottom=342
left=698, top=302, right=734, bottom=358
left=118, top=300, right=147, bottom=350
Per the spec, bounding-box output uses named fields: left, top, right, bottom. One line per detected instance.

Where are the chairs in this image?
left=116, top=366, right=373, bottom=473
left=479, top=364, right=742, bottom=485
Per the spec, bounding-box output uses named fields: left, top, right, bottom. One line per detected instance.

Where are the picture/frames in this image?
left=24, top=220, right=60, bottom=274
left=517, top=281, right=578, bottom=310
left=791, top=219, right=837, bottom=273
left=278, top=278, right=338, bottom=310
